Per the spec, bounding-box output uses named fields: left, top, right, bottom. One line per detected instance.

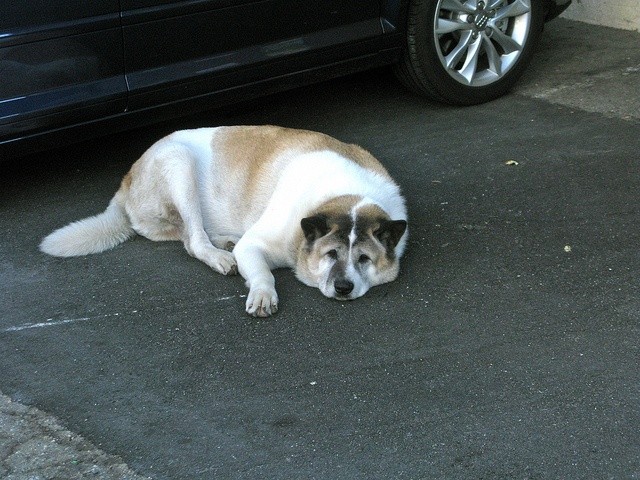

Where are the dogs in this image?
left=37, top=125, right=410, bottom=319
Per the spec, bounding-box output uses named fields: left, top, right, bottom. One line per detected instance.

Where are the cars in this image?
left=0, top=0, right=576, bottom=161
